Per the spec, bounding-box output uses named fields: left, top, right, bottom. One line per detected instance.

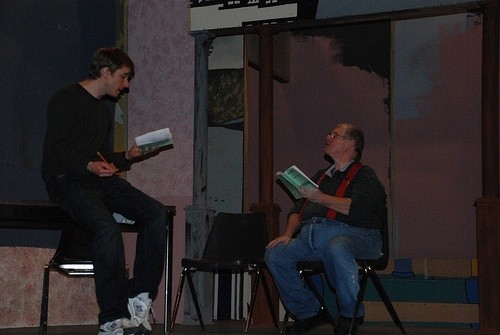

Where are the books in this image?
left=276, top=165, right=319, bottom=199
left=134, top=128, right=173, bottom=148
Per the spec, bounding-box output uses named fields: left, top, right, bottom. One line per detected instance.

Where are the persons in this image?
left=42, top=48, right=168, bottom=335
left=264, top=123, right=389, bottom=335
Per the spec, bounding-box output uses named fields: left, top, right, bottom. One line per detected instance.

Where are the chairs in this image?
left=38, top=216, right=131, bottom=335
left=279, top=190, right=406, bottom=335
left=171, top=212, right=278, bottom=334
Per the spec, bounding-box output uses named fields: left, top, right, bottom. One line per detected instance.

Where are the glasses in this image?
left=327, top=132, right=345, bottom=139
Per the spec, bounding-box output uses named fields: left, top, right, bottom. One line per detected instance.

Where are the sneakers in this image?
left=127, top=292, right=153, bottom=331
left=98, top=317, right=142, bottom=335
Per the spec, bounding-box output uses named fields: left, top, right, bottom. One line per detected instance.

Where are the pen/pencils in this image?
left=97, top=151, right=109, bottom=165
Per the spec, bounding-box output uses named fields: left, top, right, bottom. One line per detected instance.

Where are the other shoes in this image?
left=286, top=307, right=329, bottom=335
left=334, top=315, right=363, bottom=335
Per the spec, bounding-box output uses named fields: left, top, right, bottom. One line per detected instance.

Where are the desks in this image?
left=0, top=204, right=176, bottom=335
